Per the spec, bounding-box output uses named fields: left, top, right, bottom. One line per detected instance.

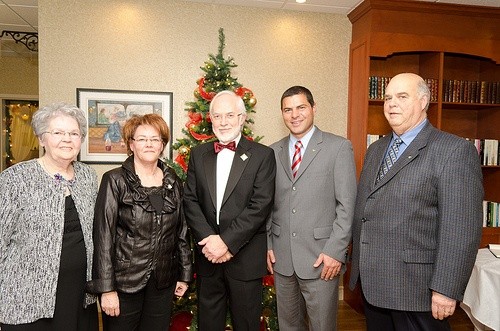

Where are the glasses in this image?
left=211, top=113, right=244, bottom=119
left=132, top=137, right=165, bottom=144
left=44, top=129, right=83, bottom=140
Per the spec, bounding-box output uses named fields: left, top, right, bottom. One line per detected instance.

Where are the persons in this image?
left=91, top=114, right=193, bottom=330
left=267, top=87, right=357, bottom=331
left=183, top=91, right=276, bottom=331
left=352, top=73, right=483, bottom=331
left=0, top=103, right=101, bottom=331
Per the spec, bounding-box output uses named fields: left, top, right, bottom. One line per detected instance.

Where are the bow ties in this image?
left=213, top=141, right=237, bottom=153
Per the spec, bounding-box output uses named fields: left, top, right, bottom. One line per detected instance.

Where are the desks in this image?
left=459, top=246, right=500, bottom=330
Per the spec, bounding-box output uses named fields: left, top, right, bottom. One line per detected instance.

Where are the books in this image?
left=366, top=75, right=500, bottom=228
left=485, top=243, right=500, bottom=259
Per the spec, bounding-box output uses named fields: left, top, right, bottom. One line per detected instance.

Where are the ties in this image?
left=291, top=142, right=303, bottom=177
left=376, top=138, right=402, bottom=185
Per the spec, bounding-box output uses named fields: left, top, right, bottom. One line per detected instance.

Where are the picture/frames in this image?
left=76, top=88, right=175, bottom=165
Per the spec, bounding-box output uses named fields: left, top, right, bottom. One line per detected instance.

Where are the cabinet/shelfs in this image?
left=338, top=1, right=500, bottom=319
